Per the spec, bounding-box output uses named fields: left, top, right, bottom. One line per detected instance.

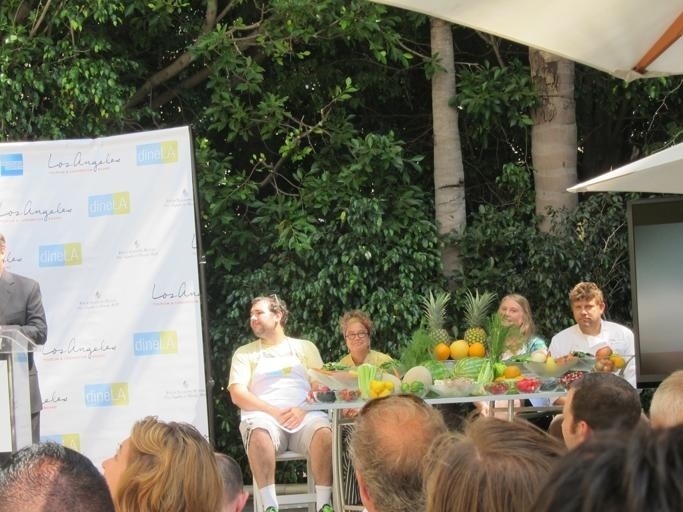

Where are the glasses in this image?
left=345, top=330, right=369, bottom=339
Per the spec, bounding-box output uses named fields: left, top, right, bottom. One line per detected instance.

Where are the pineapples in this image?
left=422, top=289, right=451, bottom=345
left=463, top=287, right=497, bottom=345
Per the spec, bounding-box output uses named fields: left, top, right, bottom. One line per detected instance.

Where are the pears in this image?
left=450, top=339, right=469, bottom=360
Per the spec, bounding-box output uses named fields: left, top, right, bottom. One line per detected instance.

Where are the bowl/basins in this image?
left=303, top=352, right=636, bottom=402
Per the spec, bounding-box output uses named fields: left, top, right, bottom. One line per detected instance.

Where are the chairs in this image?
left=251, top=450, right=318, bottom=512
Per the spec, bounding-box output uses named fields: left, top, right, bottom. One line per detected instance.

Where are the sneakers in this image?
left=266, top=507, right=275, bottom=512
left=319, top=504, right=334, bottom=512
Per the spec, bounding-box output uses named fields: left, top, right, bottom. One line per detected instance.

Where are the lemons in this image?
left=368, top=379, right=394, bottom=401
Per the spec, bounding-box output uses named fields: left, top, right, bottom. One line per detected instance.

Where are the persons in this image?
left=0, top=443, right=115, bottom=511
left=0, top=235, right=48, bottom=450
left=472, top=295, right=550, bottom=417
left=215, top=453, right=250, bottom=512
left=228, top=293, right=334, bottom=512
left=546, top=281, right=650, bottom=436
left=329, top=310, right=399, bottom=505
left=102, top=417, right=226, bottom=511
left=353, top=368, right=683, bottom=512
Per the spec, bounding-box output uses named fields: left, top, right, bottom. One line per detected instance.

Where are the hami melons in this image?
left=402, top=365, right=432, bottom=399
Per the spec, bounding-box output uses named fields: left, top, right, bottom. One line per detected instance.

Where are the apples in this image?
left=596, top=346, right=625, bottom=372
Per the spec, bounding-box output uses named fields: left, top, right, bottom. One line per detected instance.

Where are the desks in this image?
left=304, top=390, right=569, bottom=512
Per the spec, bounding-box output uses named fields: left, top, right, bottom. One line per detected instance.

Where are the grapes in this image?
left=560, top=371, right=583, bottom=389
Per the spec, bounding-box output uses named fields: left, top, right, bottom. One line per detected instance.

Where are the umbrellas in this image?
left=374, top=0, right=682, bottom=83
left=564, top=142, right=682, bottom=194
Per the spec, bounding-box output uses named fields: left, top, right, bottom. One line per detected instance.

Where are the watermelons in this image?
left=452, top=356, right=485, bottom=382
left=420, top=359, right=449, bottom=385
